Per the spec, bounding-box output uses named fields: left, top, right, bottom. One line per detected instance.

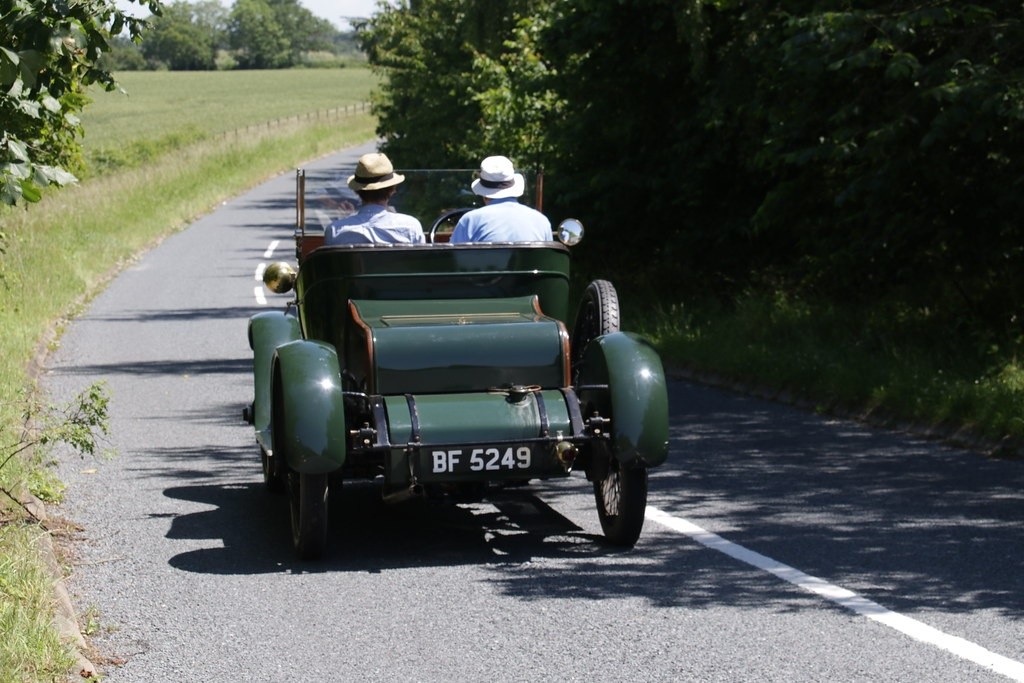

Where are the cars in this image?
left=244, top=162, right=669, bottom=555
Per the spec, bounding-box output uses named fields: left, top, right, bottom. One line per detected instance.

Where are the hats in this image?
left=347, top=153, right=405, bottom=190
left=471, top=156, right=525, bottom=200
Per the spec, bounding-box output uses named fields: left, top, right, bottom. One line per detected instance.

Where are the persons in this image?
left=323, top=153, right=426, bottom=246
left=446, top=156, right=553, bottom=241
